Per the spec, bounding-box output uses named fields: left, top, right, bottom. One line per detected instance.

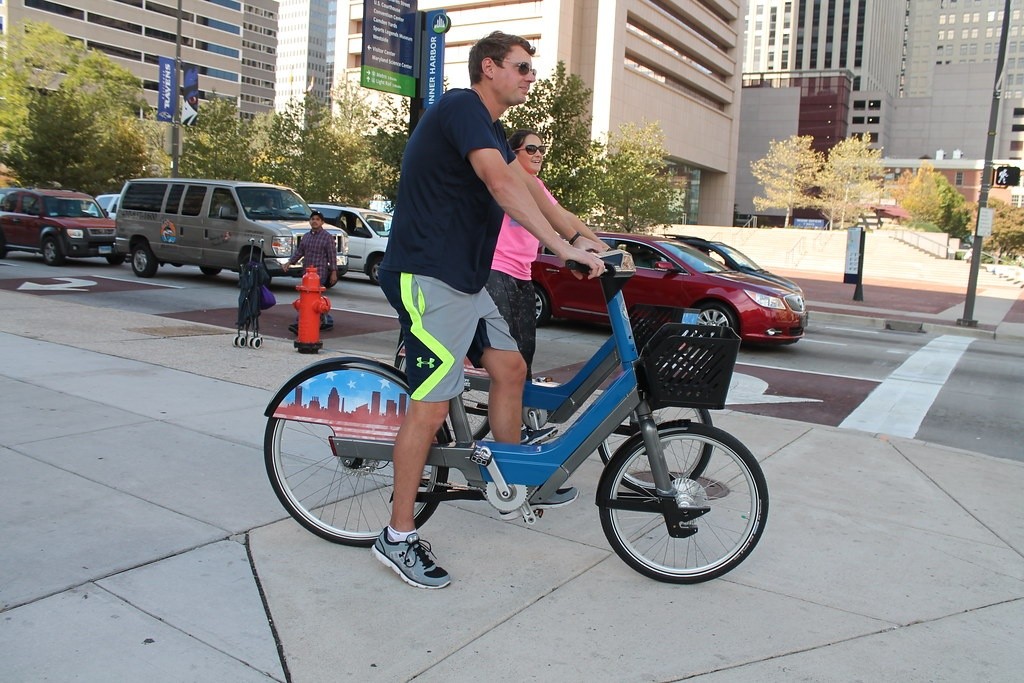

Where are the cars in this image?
left=531, top=232, right=808, bottom=345
left=89, top=194, right=121, bottom=219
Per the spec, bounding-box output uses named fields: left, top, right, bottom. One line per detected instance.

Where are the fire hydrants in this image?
left=293, top=265, right=331, bottom=353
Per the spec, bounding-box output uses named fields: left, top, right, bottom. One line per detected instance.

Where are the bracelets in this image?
left=569, top=231, right=581, bottom=245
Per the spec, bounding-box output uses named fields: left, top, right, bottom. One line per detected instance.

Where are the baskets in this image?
left=629, top=304, right=701, bottom=356
left=640, top=323, right=741, bottom=410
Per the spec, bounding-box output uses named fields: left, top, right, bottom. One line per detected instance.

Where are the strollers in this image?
left=233, top=238, right=276, bottom=349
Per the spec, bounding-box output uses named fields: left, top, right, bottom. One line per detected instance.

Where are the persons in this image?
left=257, top=196, right=278, bottom=211
left=372, top=31, right=607, bottom=589
left=485, top=128, right=613, bottom=446
left=282, top=211, right=337, bottom=335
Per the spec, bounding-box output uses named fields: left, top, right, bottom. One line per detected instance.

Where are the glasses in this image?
left=512, top=145, right=546, bottom=155
left=492, top=57, right=537, bottom=76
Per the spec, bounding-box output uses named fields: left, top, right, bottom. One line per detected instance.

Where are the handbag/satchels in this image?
left=261, top=285, right=276, bottom=310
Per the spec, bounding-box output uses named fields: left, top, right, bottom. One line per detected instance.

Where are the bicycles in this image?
left=263, top=247, right=770, bottom=586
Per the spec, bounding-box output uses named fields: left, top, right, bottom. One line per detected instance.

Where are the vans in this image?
left=114, top=179, right=349, bottom=288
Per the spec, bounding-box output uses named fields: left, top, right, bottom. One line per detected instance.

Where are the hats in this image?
left=310, top=211, right=324, bottom=221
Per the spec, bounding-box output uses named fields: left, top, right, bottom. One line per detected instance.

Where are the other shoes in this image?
left=319, top=324, right=333, bottom=331
left=288, top=323, right=299, bottom=336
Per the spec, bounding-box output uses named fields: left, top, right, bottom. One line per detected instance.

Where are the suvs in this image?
left=287, top=201, right=393, bottom=284
left=0, top=187, right=127, bottom=266
left=657, top=234, right=806, bottom=312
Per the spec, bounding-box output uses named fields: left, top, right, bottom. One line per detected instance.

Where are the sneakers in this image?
left=521, top=423, right=559, bottom=446
left=499, top=486, right=579, bottom=520
left=370, top=526, right=451, bottom=589
